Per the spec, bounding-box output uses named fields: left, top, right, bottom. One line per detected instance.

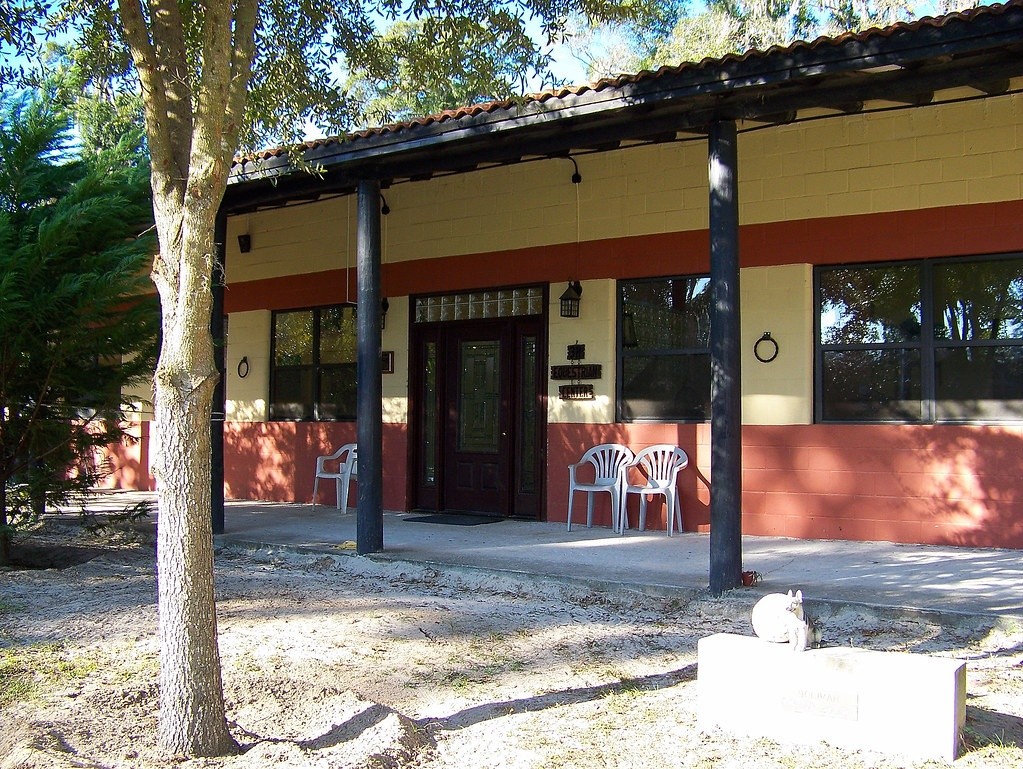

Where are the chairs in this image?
left=312, top=443, right=358, bottom=514
left=567, top=443, right=633, bottom=534
left=619, top=444, right=688, bottom=537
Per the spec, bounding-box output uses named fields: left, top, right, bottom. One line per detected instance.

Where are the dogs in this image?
left=785, top=589, right=827, bottom=654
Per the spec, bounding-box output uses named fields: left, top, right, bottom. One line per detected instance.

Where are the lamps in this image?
left=380, top=177, right=391, bottom=214
left=545, top=141, right=581, bottom=182
left=559, top=280, right=583, bottom=317
